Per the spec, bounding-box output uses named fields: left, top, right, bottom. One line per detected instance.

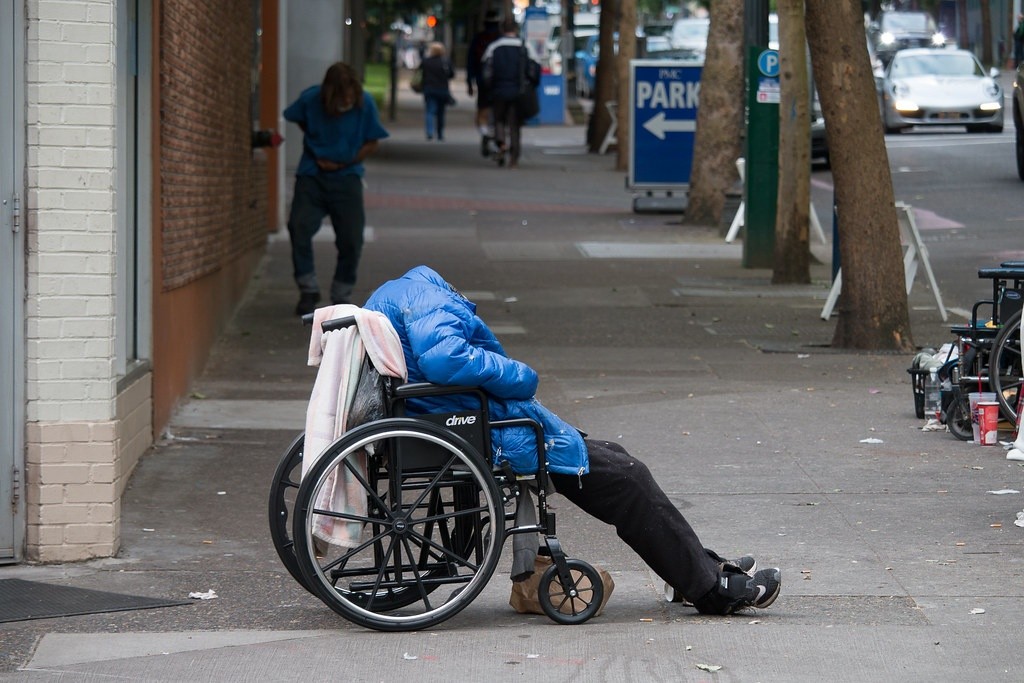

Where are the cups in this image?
left=977, top=402, right=1000, bottom=446
left=968, top=392, right=997, bottom=445
left=663, top=582, right=684, bottom=602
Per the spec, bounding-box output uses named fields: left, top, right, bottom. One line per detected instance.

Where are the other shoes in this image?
left=481, top=135, right=491, bottom=157
left=295, top=288, right=322, bottom=316
left=495, top=143, right=508, bottom=167
left=509, top=158, right=519, bottom=169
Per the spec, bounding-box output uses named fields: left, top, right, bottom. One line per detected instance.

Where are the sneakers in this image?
left=717, top=555, right=757, bottom=578
left=715, top=567, right=782, bottom=615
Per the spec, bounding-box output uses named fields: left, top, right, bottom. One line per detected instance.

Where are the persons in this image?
left=465, top=18, right=542, bottom=168
left=283, top=62, right=390, bottom=315
left=362, top=264, right=781, bottom=617
left=1013, top=14, right=1024, bottom=69
left=417, top=42, right=454, bottom=140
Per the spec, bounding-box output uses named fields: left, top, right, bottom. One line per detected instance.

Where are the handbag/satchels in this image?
left=410, top=68, right=423, bottom=93
left=346, top=354, right=389, bottom=454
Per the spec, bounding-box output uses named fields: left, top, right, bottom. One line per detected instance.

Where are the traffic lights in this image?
left=426, top=15, right=437, bottom=27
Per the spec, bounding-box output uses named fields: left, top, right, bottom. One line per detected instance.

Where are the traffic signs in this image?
left=628, top=58, right=704, bottom=191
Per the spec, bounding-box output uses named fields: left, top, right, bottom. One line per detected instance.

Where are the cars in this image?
left=644, top=14, right=887, bottom=168
left=809, top=12, right=1024, bottom=179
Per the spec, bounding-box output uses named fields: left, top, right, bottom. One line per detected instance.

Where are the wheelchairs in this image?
left=269, top=304, right=605, bottom=632
left=937, top=261, right=1024, bottom=440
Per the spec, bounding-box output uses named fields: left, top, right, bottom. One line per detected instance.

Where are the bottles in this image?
left=925, top=367, right=942, bottom=422
